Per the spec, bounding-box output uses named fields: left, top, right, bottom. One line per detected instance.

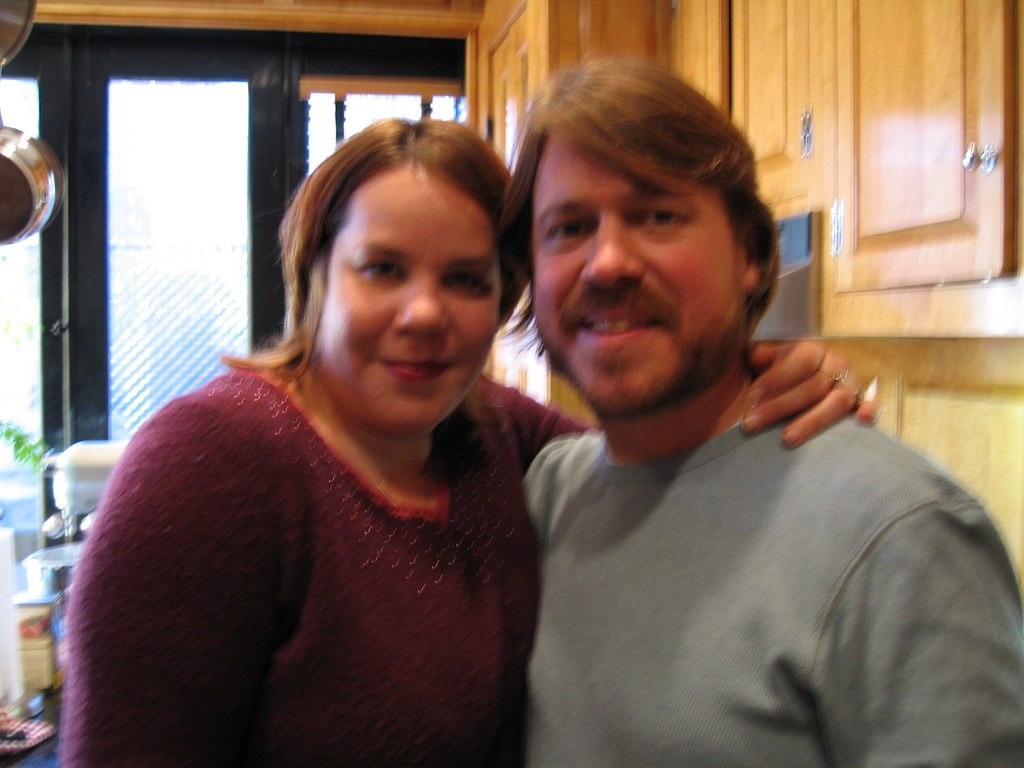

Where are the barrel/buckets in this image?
left=20, top=545, right=82, bottom=642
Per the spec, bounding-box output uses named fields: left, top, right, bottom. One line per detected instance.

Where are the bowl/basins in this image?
left=1, top=0, right=35, bottom=64
left=1, top=123, right=62, bottom=247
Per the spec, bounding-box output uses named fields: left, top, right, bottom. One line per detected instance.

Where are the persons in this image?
left=57, top=117, right=881, bottom=766
left=496, top=58, right=1024, bottom=767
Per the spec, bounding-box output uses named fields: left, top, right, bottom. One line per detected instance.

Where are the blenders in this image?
left=22, top=442, right=122, bottom=690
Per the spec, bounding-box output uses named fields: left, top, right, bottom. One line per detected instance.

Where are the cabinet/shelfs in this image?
left=456, top=0, right=1024, bottom=351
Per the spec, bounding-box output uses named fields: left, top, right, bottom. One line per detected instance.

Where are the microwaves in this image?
left=751, top=212, right=817, bottom=340
